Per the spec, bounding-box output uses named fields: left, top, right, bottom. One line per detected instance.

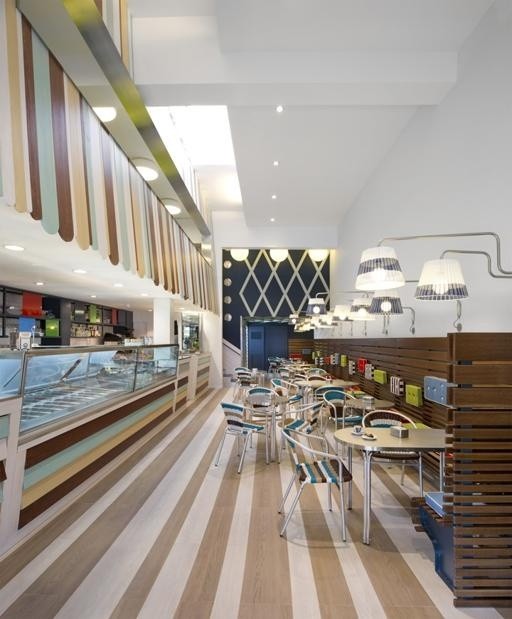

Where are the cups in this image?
left=354, top=425, right=361, bottom=433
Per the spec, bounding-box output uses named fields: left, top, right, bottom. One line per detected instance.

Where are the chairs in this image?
left=213, top=354, right=424, bottom=543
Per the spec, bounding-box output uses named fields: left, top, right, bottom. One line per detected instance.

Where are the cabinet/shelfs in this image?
left=0, top=338, right=178, bottom=557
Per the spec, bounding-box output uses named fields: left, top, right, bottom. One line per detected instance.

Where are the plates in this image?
left=362, top=433, right=377, bottom=440
left=352, top=431, right=365, bottom=435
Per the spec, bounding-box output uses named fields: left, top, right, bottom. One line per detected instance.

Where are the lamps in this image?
left=289, top=230, right=510, bottom=333
left=230, top=248, right=330, bottom=262
left=132, top=155, right=182, bottom=220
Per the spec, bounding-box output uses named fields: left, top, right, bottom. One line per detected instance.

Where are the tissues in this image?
left=390, top=422, right=408, bottom=438
